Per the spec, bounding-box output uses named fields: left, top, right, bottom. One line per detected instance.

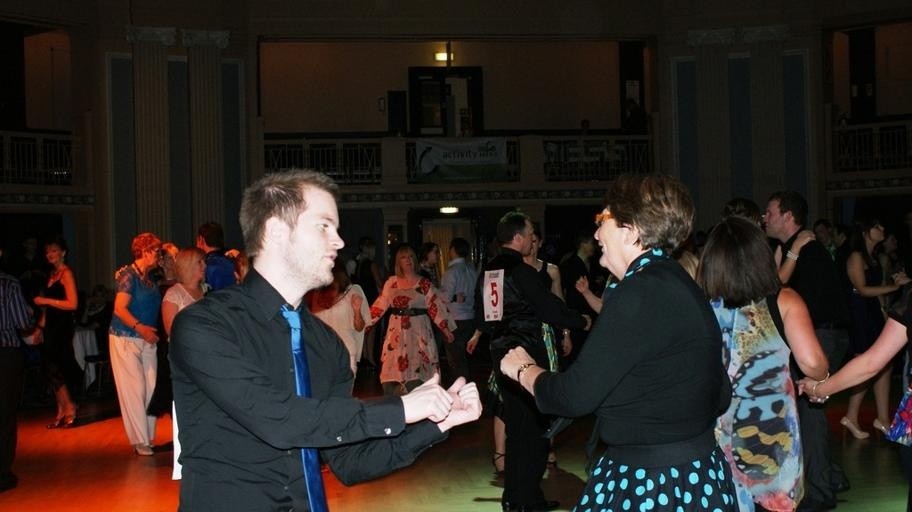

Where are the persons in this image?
left=1, top=242, right=38, bottom=493
left=31, top=238, right=78, bottom=431
left=497, top=163, right=743, bottom=511
left=761, top=192, right=851, bottom=511
left=303, top=233, right=482, bottom=424
left=166, top=167, right=485, bottom=511
left=471, top=211, right=593, bottom=511
left=541, top=234, right=614, bottom=368
left=694, top=213, right=830, bottom=512
left=78, top=283, right=113, bottom=353
left=673, top=200, right=912, bottom=441
left=109, top=221, right=250, bottom=454
left=793, top=277, right=912, bottom=512
left=463, top=219, right=573, bottom=478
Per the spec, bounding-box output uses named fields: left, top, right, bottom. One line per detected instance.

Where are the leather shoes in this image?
left=136, top=445, right=153, bottom=455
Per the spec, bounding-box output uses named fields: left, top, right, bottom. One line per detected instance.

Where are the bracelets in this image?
left=36, top=325, right=45, bottom=331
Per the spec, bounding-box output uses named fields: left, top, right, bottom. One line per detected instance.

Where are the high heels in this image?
left=63, top=404, right=79, bottom=428
left=47, top=415, right=64, bottom=429
left=873, top=418, right=891, bottom=439
left=840, top=417, right=869, bottom=439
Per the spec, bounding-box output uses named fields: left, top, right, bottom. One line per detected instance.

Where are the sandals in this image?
left=492, top=452, right=505, bottom=475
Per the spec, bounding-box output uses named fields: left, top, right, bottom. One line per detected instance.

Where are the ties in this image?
left=279, top=305, right=329, bottom=511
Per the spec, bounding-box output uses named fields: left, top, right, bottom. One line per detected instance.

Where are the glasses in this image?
left=595, top=213, right=613, bottom=226
